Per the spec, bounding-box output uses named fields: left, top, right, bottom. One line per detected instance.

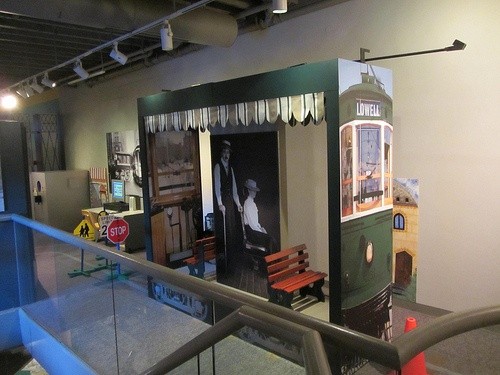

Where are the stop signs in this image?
left=107, top=218, right=129, bottom=244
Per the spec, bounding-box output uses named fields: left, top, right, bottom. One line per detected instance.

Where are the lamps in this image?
left=73, top=59, right=89, bottom=79
left=109, top=42, right=128, bottom=65
left=16, top=72, right=56, bottom=100
left=160, top=20, right=173, bottom=50
left=272, top=0, right=288, bottom=14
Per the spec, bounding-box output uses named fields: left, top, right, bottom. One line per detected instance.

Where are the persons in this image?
left=242, top=179, right=282, bottom=264
left=213, top=141, right=243, bottom=258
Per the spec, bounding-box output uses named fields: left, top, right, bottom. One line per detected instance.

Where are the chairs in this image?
left=241, top=213, right=265, bottom=272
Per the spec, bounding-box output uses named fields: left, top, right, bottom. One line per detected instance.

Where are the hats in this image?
left=220, top=139, right=233, bottom=151
left=245, top=179, right=260, bottom=192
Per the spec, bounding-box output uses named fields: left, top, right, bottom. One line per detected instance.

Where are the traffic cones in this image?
left=403, top=318, right=427, bottom=375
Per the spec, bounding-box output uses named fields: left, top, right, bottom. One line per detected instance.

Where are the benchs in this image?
left=183, top=237, right=214, bottom=279
left=265, top=245, right=328, bottom=312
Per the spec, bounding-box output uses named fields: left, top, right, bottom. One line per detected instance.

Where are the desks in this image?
left=83, top=208, right=144, bottom=251
